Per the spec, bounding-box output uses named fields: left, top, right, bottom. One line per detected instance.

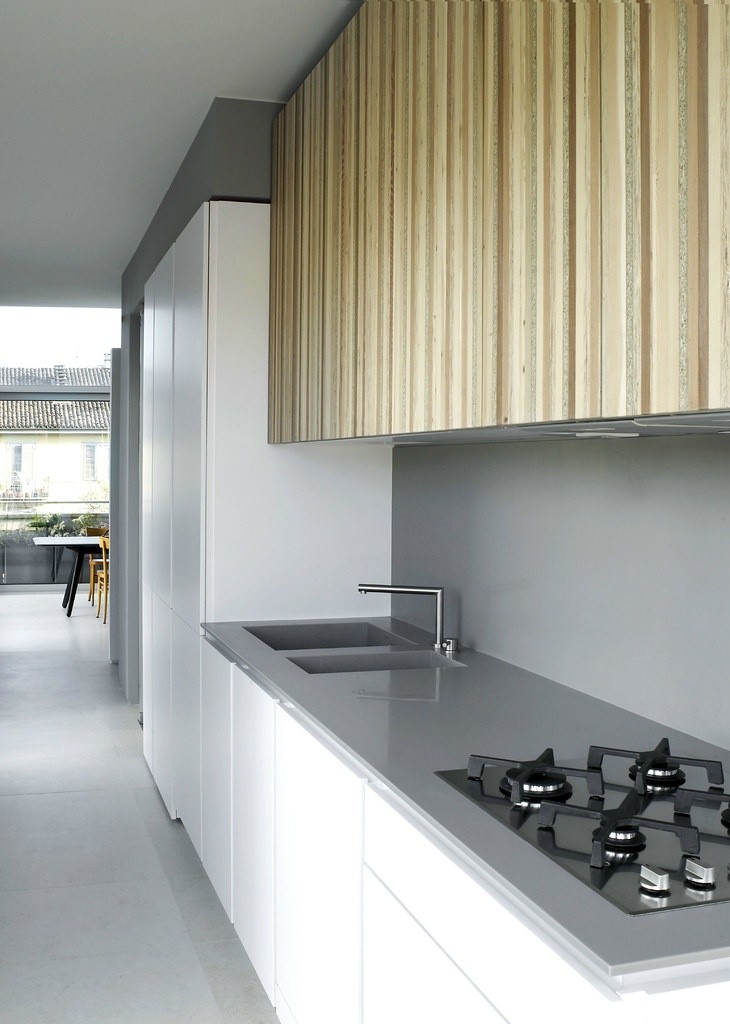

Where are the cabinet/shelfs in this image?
left=265, top=0, right=730, bottom=448
left=200, top=640, right=730, bottom=1024
left=141, top=200, right=391, bottom=822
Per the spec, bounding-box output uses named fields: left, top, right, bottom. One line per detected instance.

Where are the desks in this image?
left=33, top=535, right=109, bottom=617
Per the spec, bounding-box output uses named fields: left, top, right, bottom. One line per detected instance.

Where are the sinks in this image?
left=240, top=620, right=420, bottom=652
left=284, top=649, right=468, bottom=675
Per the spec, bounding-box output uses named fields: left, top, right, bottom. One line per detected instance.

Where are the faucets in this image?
left=357, top=583, right=444, bottom=649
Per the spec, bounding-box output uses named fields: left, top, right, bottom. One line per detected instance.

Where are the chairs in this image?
left=86, top=526, right=110, bottom=606
left=96, top=537, right=109, bottom=624
